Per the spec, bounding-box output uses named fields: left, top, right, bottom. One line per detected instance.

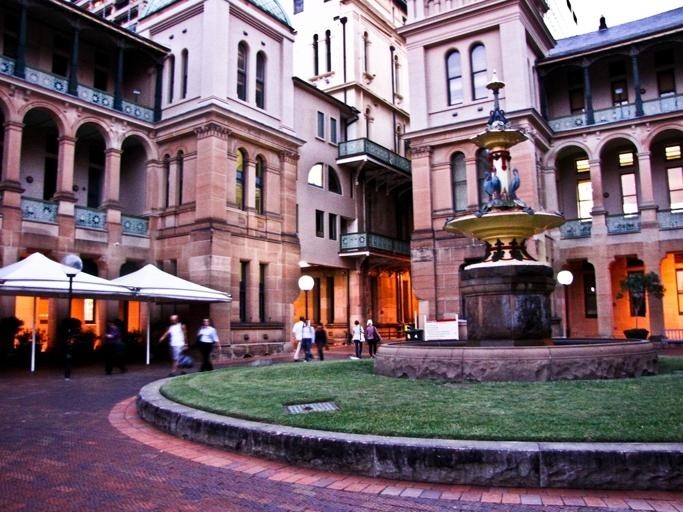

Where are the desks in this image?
left=404, top=329, right=423, bottom=341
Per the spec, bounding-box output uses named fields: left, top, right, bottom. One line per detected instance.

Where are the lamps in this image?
left=131, top=87, right=141, bottom=96
left=599, top=16, right=607, bottom=32
left=355, top=176, right=360, bottom=186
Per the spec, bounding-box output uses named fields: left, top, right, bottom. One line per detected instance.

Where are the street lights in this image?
left=556, top=270, right=573, bottom=339
left=297, top=275, right=314, bottom=321
left=60, top=254, right=83, bottom=381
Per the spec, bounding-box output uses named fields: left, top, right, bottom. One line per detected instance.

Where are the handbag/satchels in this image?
left=177, top=355, right=193, bottom=368
left=373, top=334, right=379, bottom=343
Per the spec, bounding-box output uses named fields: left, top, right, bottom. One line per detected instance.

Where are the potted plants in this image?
left=612, top=270, right=666, bottom=340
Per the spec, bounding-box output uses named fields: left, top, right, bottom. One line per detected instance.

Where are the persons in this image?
left=291, top=315, right=305, bottom=362
left=300, top=319, right=315, bottom=363
left=101, top=317, right=130, bottom=376
left=157, top=314, right=188, bottom=376
left=61, top=318, right=84, bottom=382
left=365, top=319, right=383, bottom=359
left=196, top=318, right=222, bottom=371
left=314, top=322, right=328, bottom=362
left=350, top=320, right=365, bottom=360
